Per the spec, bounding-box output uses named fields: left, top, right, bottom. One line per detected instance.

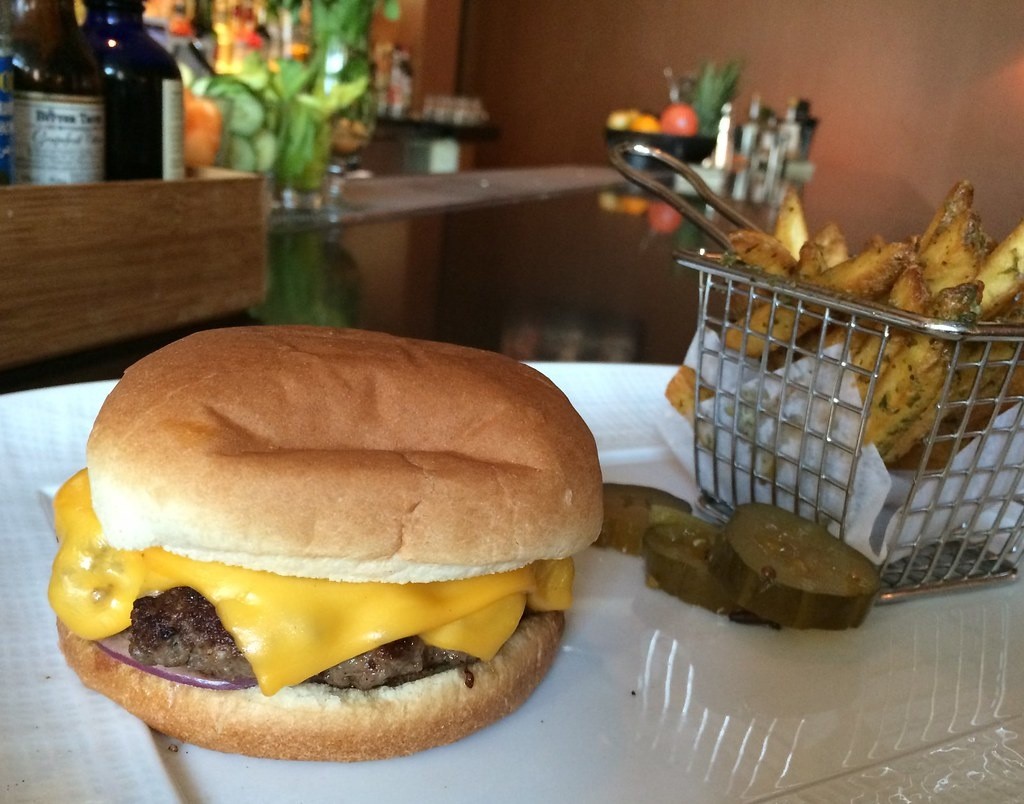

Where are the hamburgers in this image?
left=46, top=327, right=604, bottom=764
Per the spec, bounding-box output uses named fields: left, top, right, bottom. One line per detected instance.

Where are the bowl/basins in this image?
left=604, top=129, right=718, bottom=173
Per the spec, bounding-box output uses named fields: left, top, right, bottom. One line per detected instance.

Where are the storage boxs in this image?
left=0, top=163, right=269, bottom=367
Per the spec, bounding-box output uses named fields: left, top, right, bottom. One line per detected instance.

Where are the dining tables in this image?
left=0, top=361, right=1024, bottom=804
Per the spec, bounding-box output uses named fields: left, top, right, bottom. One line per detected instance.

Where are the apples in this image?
left=662, top=104, right=698, bottom=136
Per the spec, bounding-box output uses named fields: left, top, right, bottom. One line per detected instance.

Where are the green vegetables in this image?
left=691, top=58, right=742, bottom=135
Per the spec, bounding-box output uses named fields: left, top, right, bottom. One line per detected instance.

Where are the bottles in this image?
left=367, top=40, right=415, bottom=117
left=0, top=0, right=16, bottom=183
left=78, top=0, right=187, bottom=181
left=13, top=0, right=107, bottom=185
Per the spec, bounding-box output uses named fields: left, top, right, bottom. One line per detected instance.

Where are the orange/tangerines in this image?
left=630, top=116, right=659, bottom=134
